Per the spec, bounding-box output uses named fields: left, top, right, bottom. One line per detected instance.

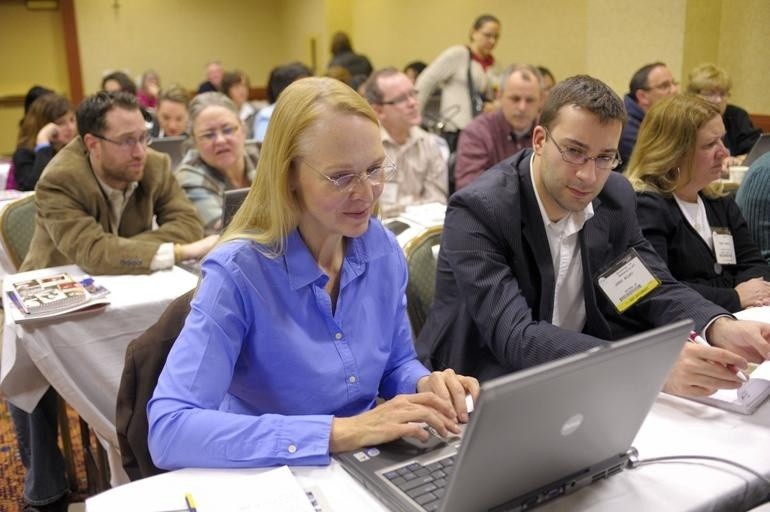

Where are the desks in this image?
left=0, top=155, right=770, bottom=511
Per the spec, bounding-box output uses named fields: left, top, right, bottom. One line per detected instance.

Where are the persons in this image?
left=7, top=86, right=221, bottom=512
left=412, top=14, right=504, bottom=156
left=414, top=75, right=770, bottom=400
left=326, top=27, right=375, bottom=77
left=1, top=55, right=770, bottom=313
left=147, top=75, right=483, bottom=472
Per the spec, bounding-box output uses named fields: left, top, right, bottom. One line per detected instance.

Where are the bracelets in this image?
left=175, top=243, right=183, bottom=264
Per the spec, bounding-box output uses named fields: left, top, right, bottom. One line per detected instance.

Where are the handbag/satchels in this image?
left=420, top=120, right=460, bottom=153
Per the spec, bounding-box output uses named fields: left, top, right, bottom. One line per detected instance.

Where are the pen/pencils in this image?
left=688, top=330, right=747, bottom=382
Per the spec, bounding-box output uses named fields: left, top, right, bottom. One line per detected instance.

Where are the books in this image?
left=681, top=358, right=770, bottom=416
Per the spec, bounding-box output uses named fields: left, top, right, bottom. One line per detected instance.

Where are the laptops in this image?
left=332, top=318, right=692, bottom=512
left=176, top=188, right=250, bottom=276
left=722, top=132, right=770, bottom=179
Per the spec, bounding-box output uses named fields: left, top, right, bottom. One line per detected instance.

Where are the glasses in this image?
left=302, top=153, right=399, bottom=193
left=197, top=122, right=242, bottom=144
left=379, top=90, right=419, bottom=105
left=94, top=133, right=152, bottom=149
left=544, top=128, right=622, bottom=170
